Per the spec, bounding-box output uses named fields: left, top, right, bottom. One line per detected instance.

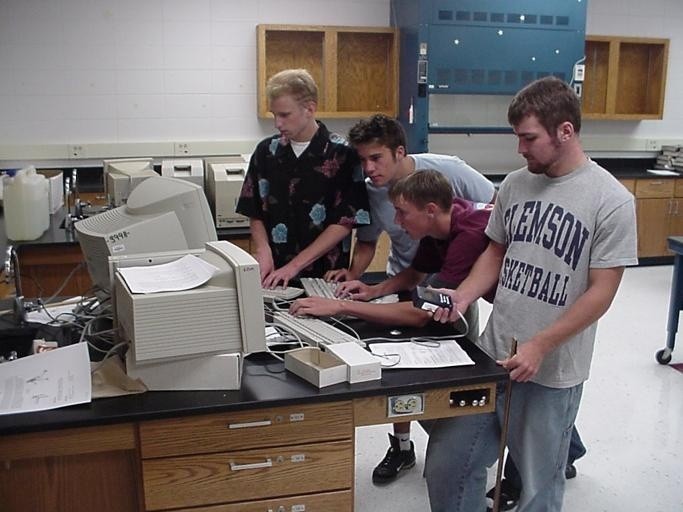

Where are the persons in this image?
left=282, top=165, right=589, bottom=512
left=232, top=65, right=373, bottom=292
left=320, top=110, right=503, bottom=487
left=418, top=72, right=642, bottom=511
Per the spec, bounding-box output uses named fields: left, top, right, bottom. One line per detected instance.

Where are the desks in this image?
left=0, top=269, right=510, bottom=511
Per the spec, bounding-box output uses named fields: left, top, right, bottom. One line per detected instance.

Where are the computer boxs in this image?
left=124, top=346, right=245, bottom=392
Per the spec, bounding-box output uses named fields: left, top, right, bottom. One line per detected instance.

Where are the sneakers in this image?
left=485, top=479, right=524, bottom=511
left=372, top=433, right=416, bottom=486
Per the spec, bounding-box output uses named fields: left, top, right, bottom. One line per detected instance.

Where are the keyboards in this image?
left=262, top=285, right=305, bottom=304
left=299, top=276, right=359, bottom=321
left=272, top=310, right=367, bottom=350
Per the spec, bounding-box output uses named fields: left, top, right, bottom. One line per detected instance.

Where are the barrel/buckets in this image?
left=26, top=164, right=51, bottom=232
left=4, top=171, right=45, bottom=242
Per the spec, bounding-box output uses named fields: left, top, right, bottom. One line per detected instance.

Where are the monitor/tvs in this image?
left=113, top=238, right=269, bottom=366
left=162, top=158, right=204, bottom=189
left=102, top=157, right=161, bottom=207
left=206, top=161, right=250, bottom=229
left=73, top=175, right=219, bottom=312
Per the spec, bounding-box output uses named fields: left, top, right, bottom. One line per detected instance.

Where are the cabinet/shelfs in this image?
left=617, top=180, right=683, bottom=259
left=254, top=22, right=402, bottom=122
left=580, top=34, right=670, bottom=121
